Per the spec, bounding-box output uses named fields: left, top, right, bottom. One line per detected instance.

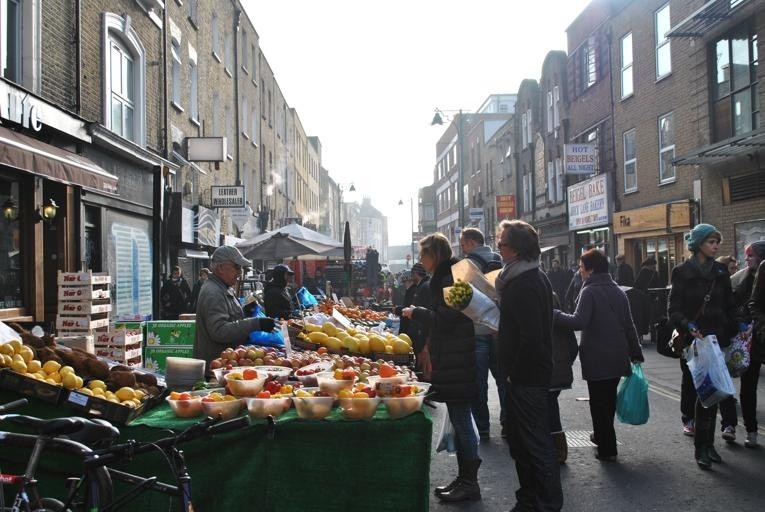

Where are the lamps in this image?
left=1, top=193, right=20, bottom=222
left=36, top=196, right=60, bottom=228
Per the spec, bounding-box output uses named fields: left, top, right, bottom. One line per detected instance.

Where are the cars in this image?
left=8, top=249, right=20, bottom=294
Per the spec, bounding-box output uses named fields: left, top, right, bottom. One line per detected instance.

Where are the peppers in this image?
left=170, top=368, right=419, bottom=417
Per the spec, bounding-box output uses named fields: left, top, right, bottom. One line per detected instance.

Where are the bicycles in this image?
left=0, top=397, right=251, bottom=512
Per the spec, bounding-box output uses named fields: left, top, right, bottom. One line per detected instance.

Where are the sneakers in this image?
left=682, top=420, right=757, bottom=467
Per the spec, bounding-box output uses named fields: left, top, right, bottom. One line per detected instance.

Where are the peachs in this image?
left=210, top=344, right=380, bottom=376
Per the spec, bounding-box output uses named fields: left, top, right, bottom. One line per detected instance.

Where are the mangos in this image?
left=299, top=322, right=411, bottom=355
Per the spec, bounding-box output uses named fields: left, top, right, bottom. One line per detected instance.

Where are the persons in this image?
left=162, top=266, right=210, bottom=320
left=679, top=242, right=765, bottom=446
left=667, top=224, right=738, bottom=467
left=379, top=227, right=659, bottom=438
left=264, top=264, right=295, bottom=320
left=548, top=291, right=579, bottom=462
left=245, top=282, right=263, bottom=305
left=310, top=268, right=324, bottom=295
left=193, top=244, right=276, bottom=382
left=402, top=233, right=482, bottom=501
left=553, top=250, right=644, bottom=459
left=485, top=219, right=565, bottom=512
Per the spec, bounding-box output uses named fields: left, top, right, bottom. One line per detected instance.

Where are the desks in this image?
left=0, top=376, right=430, bottom=512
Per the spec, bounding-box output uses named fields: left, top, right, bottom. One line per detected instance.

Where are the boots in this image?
left=550, top=432, right=567, bottom=462
left=436, top=460, right=481, bottom=500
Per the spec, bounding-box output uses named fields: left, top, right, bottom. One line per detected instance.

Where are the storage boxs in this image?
left=110, top=311, right=199, bottom=376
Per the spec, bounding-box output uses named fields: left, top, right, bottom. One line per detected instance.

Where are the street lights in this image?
left=430, top=107, right=464, bottom=234
left=336, top=182, right=357, bottom=240
left=398, top=196, right=415, bottom=266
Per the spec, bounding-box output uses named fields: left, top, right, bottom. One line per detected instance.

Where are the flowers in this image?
left=444, top=277, right=500, bottom=331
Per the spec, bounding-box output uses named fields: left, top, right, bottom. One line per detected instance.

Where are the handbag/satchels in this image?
left=656, top=321, right=692, bottom=358
left=723, top=321, right=754, bottom=377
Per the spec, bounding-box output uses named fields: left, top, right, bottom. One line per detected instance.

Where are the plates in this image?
left=165, top=356, right=207, bottom=388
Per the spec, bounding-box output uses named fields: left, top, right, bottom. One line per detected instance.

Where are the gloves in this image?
left=259, top=318, right=275, bottom=332
left=243, top=301, right=259, bottom=317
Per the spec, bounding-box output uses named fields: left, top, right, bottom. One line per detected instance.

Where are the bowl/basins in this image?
left=165, top=362, right=432, bottom=421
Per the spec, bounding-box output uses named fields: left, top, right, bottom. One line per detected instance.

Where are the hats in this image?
left=275, top=264, right=295, bottom=275
left=685, top=224, right=716, bottom=251
left=212, top=246, right=251, bottom=266
left=412, top=263, right=426, bottom=276
left=752, top=240, right=765, bottom=257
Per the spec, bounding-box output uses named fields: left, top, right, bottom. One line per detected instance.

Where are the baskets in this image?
left=373, top=352, right=409, bottom=365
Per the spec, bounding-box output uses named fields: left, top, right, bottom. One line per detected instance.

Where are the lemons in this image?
left=0, top=340, right=147, bottom=409
